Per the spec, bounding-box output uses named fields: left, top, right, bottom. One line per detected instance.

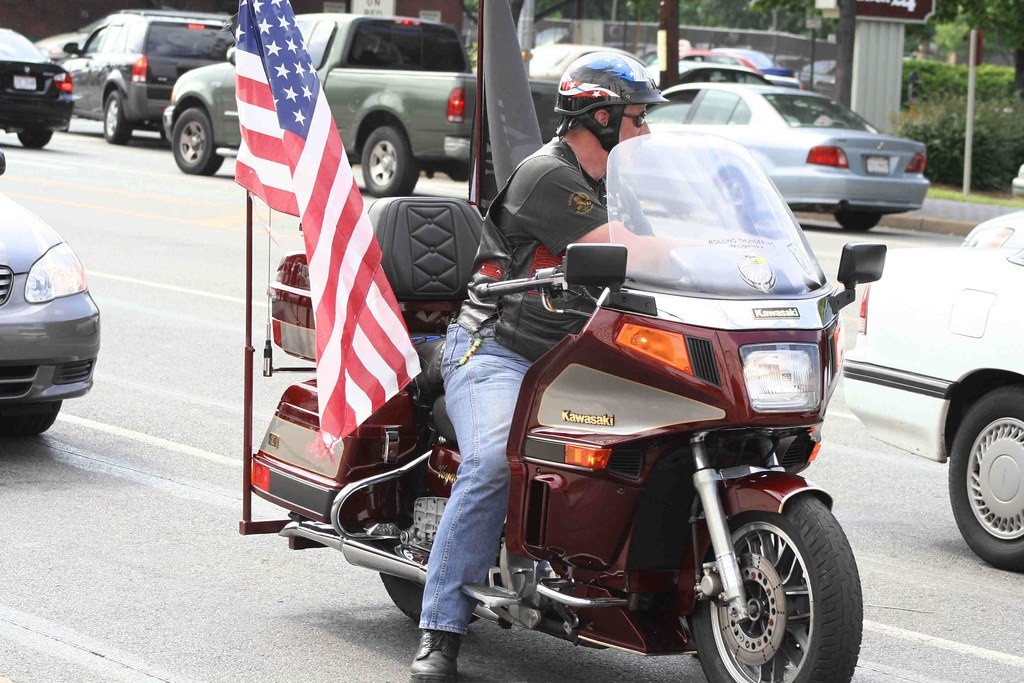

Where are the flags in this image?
left=233, top=0, right=427, bottom=456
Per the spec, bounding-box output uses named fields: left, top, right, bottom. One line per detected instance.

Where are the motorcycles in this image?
left=201, top=0, right=864, bottom=682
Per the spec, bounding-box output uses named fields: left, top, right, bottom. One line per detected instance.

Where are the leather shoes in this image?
left=409, top=629, right=461, bottom=683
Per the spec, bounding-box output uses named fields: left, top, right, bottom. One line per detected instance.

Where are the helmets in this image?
left=553, top=49, right=670, bottom=116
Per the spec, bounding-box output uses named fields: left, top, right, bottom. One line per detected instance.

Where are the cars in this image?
left=0, top=159, right=100, bottom=443
left=840, top=204, right=1024, bottom=573
left=643, top=84, right=929, bottom=226
left=53, top=9, right=233, bottom=146
left=519, top=28, right=799, bottom=158
left=1, top=27, right=75, bottom=150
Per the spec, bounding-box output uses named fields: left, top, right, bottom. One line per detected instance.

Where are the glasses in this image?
left=592, top=110, right=647, bottom=128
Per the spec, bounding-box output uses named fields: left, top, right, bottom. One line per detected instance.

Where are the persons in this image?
left=411, top=47, right=691, bottom=683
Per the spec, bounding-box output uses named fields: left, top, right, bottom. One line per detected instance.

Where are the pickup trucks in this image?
left=157, top=8, right=567, bottom=203
